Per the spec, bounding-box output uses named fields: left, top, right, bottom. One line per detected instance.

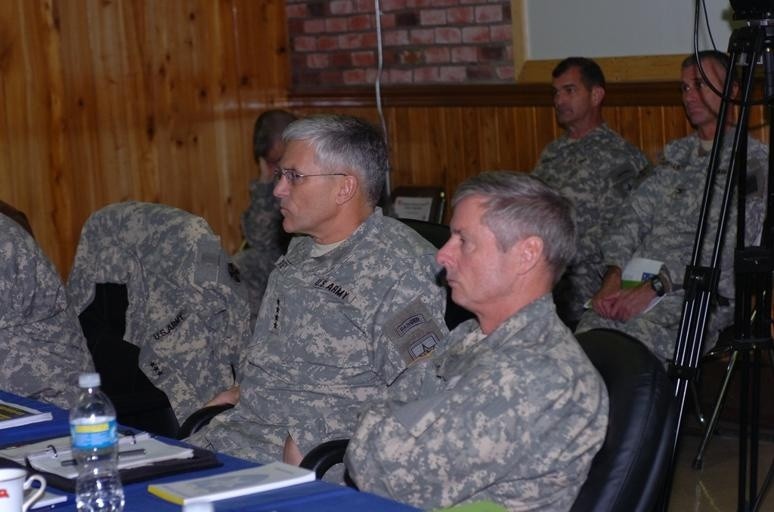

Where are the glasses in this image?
left=272, top=164, right=348, bottom=187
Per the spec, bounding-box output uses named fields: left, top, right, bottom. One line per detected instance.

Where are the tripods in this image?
left=672, top=24, right=773, bottom=511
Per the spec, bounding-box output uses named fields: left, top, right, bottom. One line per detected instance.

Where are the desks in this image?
left=0, top=388, right=423, bottom=512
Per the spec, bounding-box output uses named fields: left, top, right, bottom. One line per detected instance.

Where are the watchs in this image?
left=651, top=276, right=667, bottom=298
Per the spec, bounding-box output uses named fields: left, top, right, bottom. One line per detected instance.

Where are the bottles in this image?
left=69, top=373, right=125, bottom=512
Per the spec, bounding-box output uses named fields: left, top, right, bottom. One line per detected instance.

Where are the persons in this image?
left=230, top=111, right=298, bottom=335
left=574, top=50, right=771, bottom=366
left=341, top=168, right=611, bottom=512
left=1, top=214, right=96, bottom=411
left=530, top=57, right=655, bottom=325
left=176, top=113, right=452, bottom=468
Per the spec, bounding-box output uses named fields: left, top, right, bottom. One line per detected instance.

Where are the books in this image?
left=0, top=428, right=195, bottom=481
left=1, top=400, right=54, bottom=430
left=147, top=461, right=316, bottom=505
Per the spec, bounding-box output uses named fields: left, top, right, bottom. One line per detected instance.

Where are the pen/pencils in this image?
left=61, top=448, right=147, bottom=466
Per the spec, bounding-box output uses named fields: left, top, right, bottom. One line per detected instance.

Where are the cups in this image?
left=0, top=468, right=47, bottom=512
